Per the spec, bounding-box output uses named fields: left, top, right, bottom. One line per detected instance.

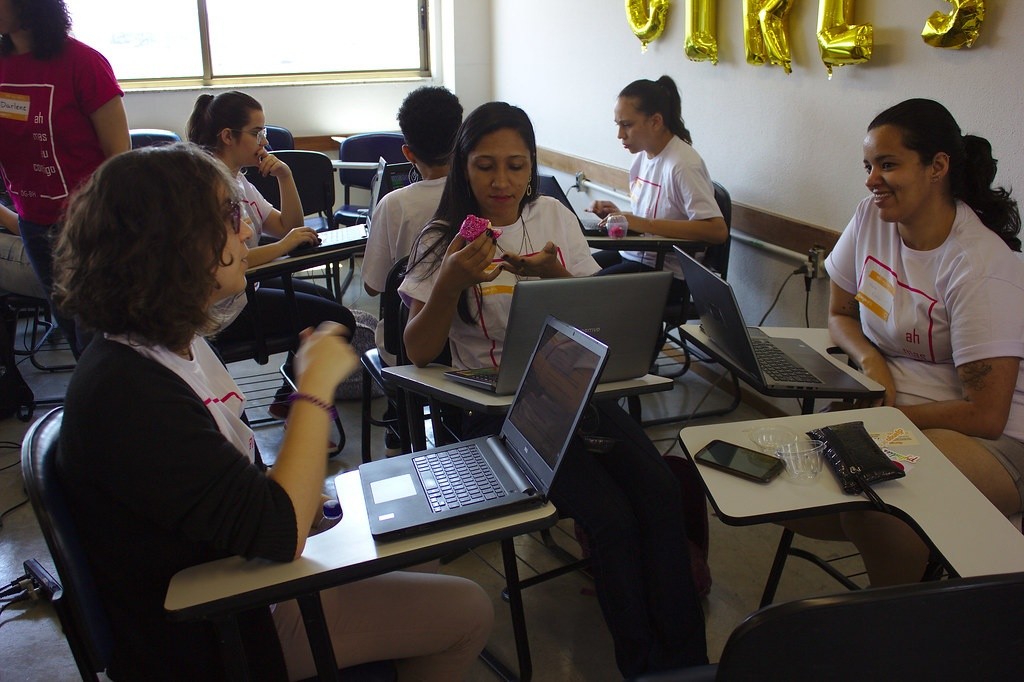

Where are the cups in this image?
left=606, top=214, right=629, bottom=240
left=748, top=428, right=798, bottom=455
left=777, top=440, right=823, bottom=480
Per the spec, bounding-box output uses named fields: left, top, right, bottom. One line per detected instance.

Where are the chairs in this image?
left=241, top=149, right=336, bottom=233
left=264, top=125, right=295, bottom=151
left=715, top=572, right=1024, bottom=682
left=399, top=298, right=462, bottom=452
left=131, top=134, right=180, bottom=151
left=333, top=133, right=423, bottom=297
left=359, top=255, right=412, bottom=463
left=21, top=406, right=398, bottom=682
left=634, top=182, right=742, bottom=427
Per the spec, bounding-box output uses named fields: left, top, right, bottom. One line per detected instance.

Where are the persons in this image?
left=0, top=0, right=131, bottom=363
left=398, top=101, right=711, bottom=682
left=362, top=87, right=463, bottom=456
left=591, top=76, right=729, bottom=305
left=56, top=141, right=495, bottom=682
left=186, top=92, right=356, bottom=453
left=771, top=98, right=1024, bottom=588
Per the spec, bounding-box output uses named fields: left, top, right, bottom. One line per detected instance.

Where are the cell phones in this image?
left=694, top=439, right=786, bottom=483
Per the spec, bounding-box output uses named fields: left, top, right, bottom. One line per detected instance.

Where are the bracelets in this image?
left=287, top=393, right=336, bottom=421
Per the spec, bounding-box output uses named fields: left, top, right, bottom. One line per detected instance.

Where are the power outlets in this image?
left=575, top=171, right=589, bottom=192
left=808, top=248, right=826, bottom=279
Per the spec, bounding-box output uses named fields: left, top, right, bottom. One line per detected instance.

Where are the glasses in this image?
left=217, top=129, right=267, bottom=145
left=220, top=200, right=241, bottom=234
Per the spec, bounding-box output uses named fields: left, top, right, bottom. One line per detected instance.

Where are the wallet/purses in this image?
left=807, top=420, right=905, bottom=510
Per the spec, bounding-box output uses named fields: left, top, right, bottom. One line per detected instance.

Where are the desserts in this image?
left=460, top=214, right=503, bottom=242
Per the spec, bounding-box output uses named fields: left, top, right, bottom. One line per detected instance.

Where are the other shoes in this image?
left=268, top=398, right=291, bottom=420
left=384, top=431, right=403, bottom=458
left=283, top=414, right=339, bottom=454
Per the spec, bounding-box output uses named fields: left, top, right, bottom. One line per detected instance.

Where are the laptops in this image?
left=288, top=155, right=388, bottom=256
left=359, top=314, right=610, bottom=542
left=671, top=243, right=871, bottom=394
left=445, top=270, right=673, bottom=396
left=533, top=175, right=644, bottom=236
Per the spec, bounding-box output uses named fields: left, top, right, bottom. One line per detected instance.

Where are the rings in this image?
left=271, top=159, right=276, bottom=163
left=518, top=266, right=524, bottom=272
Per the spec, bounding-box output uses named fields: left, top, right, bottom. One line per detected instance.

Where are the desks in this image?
left=379, top=363, right=675, bottom=583
left=159, top=468, right=562, bottom=682
left=243, top=242, right=368, bottom=286
left=581, top=230, right=706, bottom=323
left=679, top=323, right=888, bottom=415
left=678, top=405, right=1024, bottom=611
